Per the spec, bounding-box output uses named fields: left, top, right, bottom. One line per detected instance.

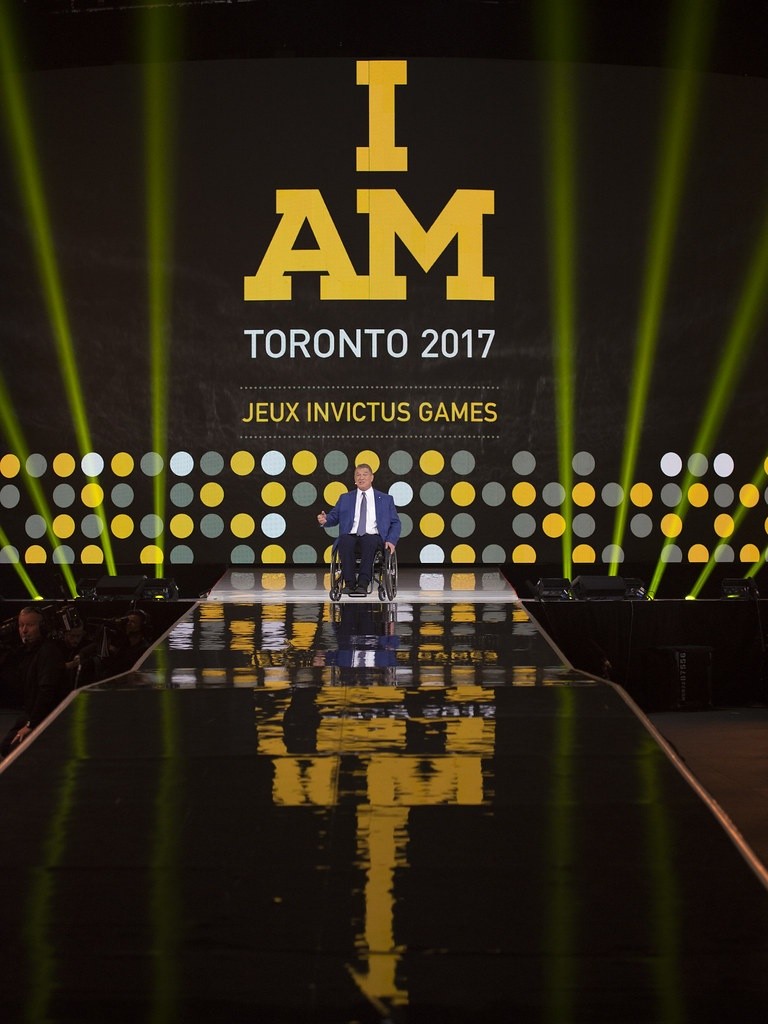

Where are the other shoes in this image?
left=340, top=585, right=353, bottom=593
left=355, top=586, right=367, bottom=593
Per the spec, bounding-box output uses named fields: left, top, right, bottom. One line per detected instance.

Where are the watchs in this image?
left=26, top=720, right=32, bottom=729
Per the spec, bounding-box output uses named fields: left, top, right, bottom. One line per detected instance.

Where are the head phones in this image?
left=139, top=610, right=152, bottom=634
left=30, top=607, right=49, bottom=635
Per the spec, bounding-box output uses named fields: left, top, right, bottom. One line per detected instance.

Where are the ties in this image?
left=357, top=491, right=367, bottom=536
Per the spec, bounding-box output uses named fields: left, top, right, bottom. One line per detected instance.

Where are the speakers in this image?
left=94, top=575, right=148, bottom=597
left=571, top=576, right=625, bottom=600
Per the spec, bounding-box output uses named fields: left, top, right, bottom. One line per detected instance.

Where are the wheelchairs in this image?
left=329, top=538, right=399, bottom=603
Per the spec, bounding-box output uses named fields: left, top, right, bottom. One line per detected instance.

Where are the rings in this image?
left=17, top=734, right=19, bottom=737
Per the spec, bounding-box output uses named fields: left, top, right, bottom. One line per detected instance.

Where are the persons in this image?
left=3, top=607, right=62, bottom=743
left=318, top=464, right=401, bottom=594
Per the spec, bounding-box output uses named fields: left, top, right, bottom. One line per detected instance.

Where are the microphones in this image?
left=24, top=638, right=32, bottom=642
left=129, top=629, right=143, bottom=635
left=355, top=483, right=357, bottom=484
